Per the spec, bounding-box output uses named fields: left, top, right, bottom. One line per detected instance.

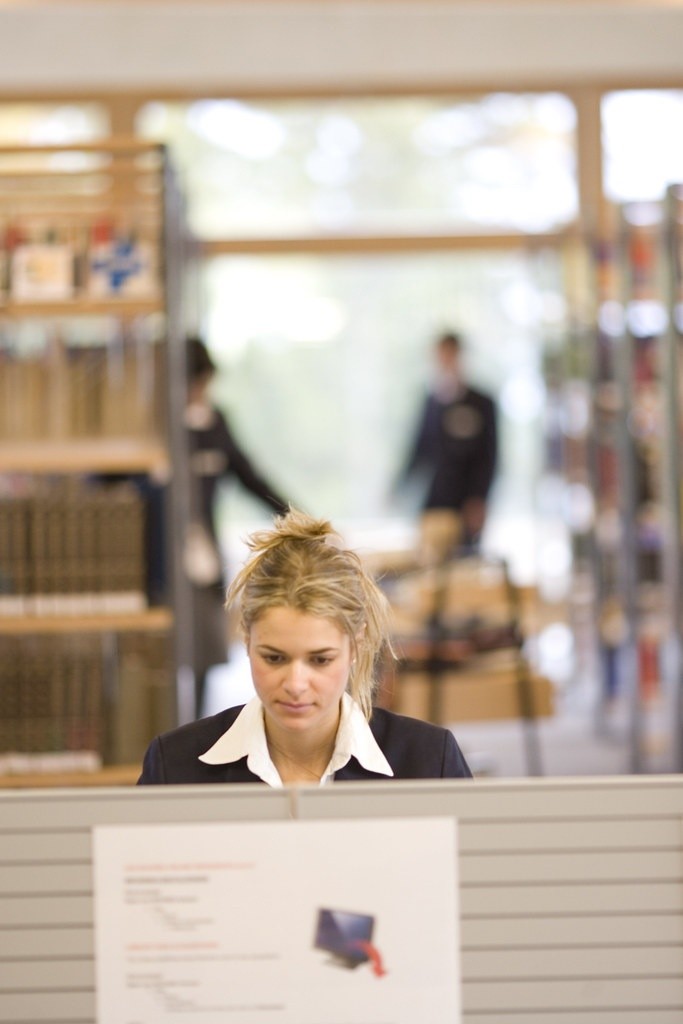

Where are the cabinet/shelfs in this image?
left=542, top=183, right=683, bottom=775
left=0, top=140, right=198, bottom=791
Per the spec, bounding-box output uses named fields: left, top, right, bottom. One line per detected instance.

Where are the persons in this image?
left=394, top=336, right=500, bottom=568
left=129, top=508, right=474, bottom=785
left=144, top=336, right=293, bottom=721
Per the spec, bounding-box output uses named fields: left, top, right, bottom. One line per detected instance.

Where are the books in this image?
left=2, top=221, right=171, bottom=770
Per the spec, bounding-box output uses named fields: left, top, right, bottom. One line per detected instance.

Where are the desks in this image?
left=326, top=516, right=565, bottom=775
